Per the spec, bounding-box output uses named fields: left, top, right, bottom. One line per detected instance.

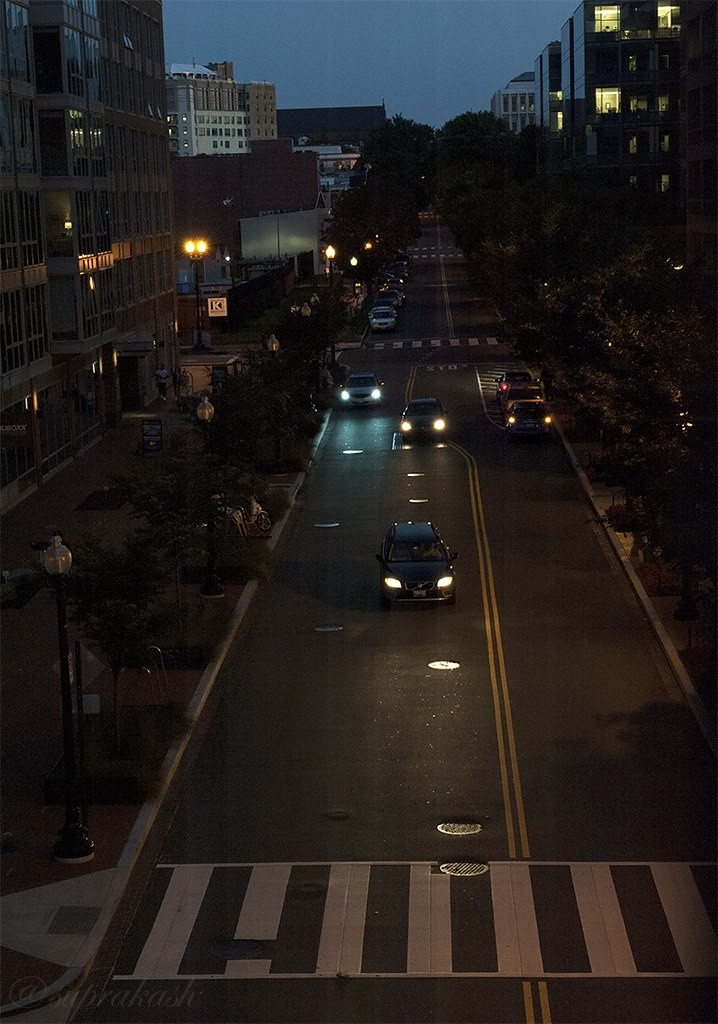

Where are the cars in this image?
left=339, top=373, right=386, bottom=409
left=377, top=521, right=458, bottom=609
left=398, top=397, right=452, bottom=445
left=501, top=399, right=555, bottom=445
left=493, top=368, right=540, bottom=409
left=367, top=251, right=414, bottom=333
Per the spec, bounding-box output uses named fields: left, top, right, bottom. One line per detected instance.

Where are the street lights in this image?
left=44, top=533, right=98, bottom=865
left=349, top=256, right=359, bottom=313
left=195, top=394, right=226, bottom=596
left=324, top=243, right=338, bottom=368
left=185, top=238, right=209, bottom=350
left=364, top=241, right=373, bottom=296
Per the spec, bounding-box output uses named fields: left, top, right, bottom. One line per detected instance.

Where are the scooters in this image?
left=208, top=492, right=272, bottom=532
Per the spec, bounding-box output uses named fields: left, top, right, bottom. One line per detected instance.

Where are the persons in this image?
left=172, top=368, right=182, bottom=400
left=423, top=543, right=442, bottom=560
left=154, top=363, right=169, bottom=401
